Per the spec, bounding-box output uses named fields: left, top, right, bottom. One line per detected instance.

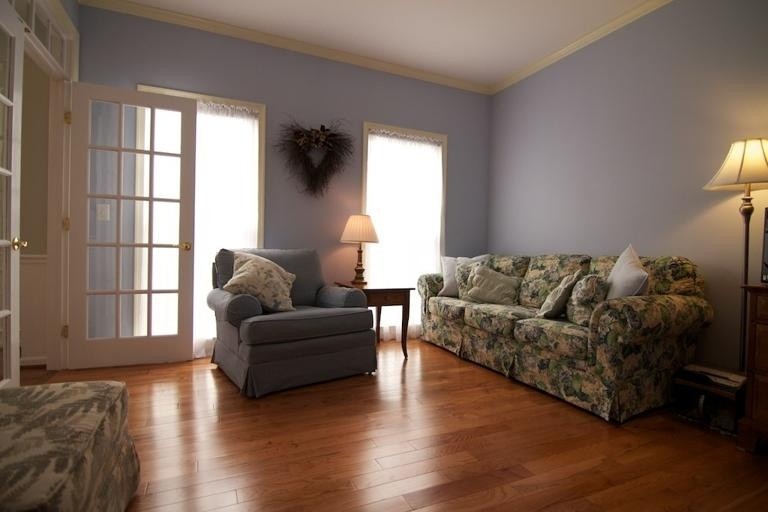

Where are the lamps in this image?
left=338, top=215, right=379, bottom=285
left=700, top=131, right=768, bottom=371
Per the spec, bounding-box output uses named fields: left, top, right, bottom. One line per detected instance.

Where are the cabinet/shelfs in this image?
left=730, top=278, right=766, bottom=454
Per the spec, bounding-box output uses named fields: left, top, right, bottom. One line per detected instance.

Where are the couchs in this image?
left=411, top=248, right=714, bottom=422
left=206, top=240, right=375, bottom=397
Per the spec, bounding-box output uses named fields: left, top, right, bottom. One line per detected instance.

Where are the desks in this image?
left=332, top=278, right=415, bottom=358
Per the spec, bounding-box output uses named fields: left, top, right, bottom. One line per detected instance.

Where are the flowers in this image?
left=271, top=115, right=358, bottom=199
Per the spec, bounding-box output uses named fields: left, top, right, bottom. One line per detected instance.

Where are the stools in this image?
left=0, top=376, right=143, bottom=511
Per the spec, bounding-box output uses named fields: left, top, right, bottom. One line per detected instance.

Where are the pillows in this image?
left=436, top=248, right=490, bottom=297
left=463, top=260, right=521, bottom=309
left=222, top=249, right=297, bottom=313
left=532, top=264, right=583, bottom=319
left=562, top=270, right=609, bottom=328
left=455, top=259, right=492, bottom=303
left=602, top=242, right=648, bottom=295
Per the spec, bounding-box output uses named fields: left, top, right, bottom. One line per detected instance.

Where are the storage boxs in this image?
left=668, top=361, right=747, bottom=437
